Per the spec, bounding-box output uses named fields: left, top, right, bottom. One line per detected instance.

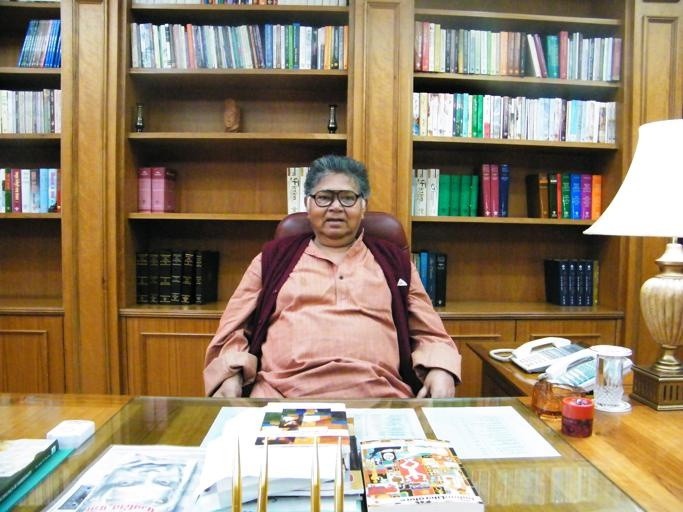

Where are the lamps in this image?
left=583, top=119, right=683, bottom=412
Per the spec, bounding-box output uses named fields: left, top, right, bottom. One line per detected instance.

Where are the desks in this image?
left=0, top=391, right=683, bottom=512
left=466, top=340, right=637, bottom=396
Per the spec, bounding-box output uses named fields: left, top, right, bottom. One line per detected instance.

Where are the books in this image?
left=17, top=17, right=62, bottom=68
left=286, top=167, right=313, bottom=215
left=530, top=169, right=604, bottom=222
left=411, top=90, right=618, bottom=144
left=129, top=18, right=350, bottom=71
left=0, top=87, right=62, bottom=135
left=0, top=166, right=61, bottom=214
left=0, top=438, right=75, bottom=512
left=411, top=161, right=510, bottom=218
left=138, top=166, right=180, bottom=212
left=133, top=0, right=348, bottom=6
left=414, top=15, right=625, bottom=80
left=412, top=247, right=449, bottom=309
left=216, top=402, right=486, bottom=511
left=134, top=246, right=221, bottom=305
left=542, top=257, right=600, bottom=307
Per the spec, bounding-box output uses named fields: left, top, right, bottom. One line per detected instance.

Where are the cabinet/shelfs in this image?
left=410, top=0, right=633, bottom=397
left=119, top=1, right=354, bottom=396
left=0, top=1, right=64, bottom=394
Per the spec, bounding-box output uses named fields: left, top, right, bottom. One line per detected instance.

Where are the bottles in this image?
left=560, top=398, right=594, bottom=438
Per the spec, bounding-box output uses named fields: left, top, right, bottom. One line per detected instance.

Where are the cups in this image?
left=593, top=354, right=631, bottom=410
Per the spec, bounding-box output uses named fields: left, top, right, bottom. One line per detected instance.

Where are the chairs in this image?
left=275, top=212, right=409, bottom=252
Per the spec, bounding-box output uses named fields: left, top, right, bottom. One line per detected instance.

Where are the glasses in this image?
left=308, top=189, right=364, bottom=208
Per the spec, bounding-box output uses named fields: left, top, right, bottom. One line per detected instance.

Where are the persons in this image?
left=201, top=155, right=463, bottom=400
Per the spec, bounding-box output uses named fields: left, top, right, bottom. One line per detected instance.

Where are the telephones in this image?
left=489, top=337, right=586, bottom=374
left=537, top=348, right=634, bottom=394
